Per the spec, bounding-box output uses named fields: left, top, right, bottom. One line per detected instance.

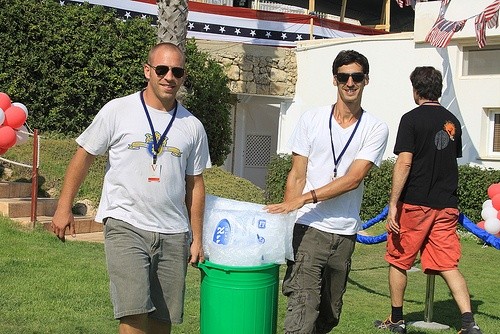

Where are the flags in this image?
left=396, top=0, right=420, bottom=11
left=425, top=0, right=500, bottom=48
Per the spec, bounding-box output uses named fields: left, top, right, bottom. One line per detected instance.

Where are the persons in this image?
left=51, top=42, right=212, bottom=334
left=263, top=50, right=389, bottom=334
left=373, top=66, right=480, bottom=334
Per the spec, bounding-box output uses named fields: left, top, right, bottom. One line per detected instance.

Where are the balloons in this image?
left=0, top=92, right=29, bottom=156
left=476, top=182, right=500, bottom=238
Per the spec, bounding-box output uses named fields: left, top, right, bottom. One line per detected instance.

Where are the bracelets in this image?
left=311, top=190, right=317, bottom=203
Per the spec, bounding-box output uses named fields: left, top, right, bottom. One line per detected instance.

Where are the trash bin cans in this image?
left=192, top=257, right=281, bottom=334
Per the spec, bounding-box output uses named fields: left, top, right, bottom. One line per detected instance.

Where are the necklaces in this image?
left=422, top=101, right=439, bottom=105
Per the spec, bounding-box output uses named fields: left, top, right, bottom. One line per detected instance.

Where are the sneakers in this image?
left=375, top=314, right=407, bottom=334
left=458, top=325, right=480, bottom=334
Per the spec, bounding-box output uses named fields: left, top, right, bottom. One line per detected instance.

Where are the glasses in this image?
left=147, top=64, right=186, bottom=78
left=336, top=72, right=366, bottom=83
